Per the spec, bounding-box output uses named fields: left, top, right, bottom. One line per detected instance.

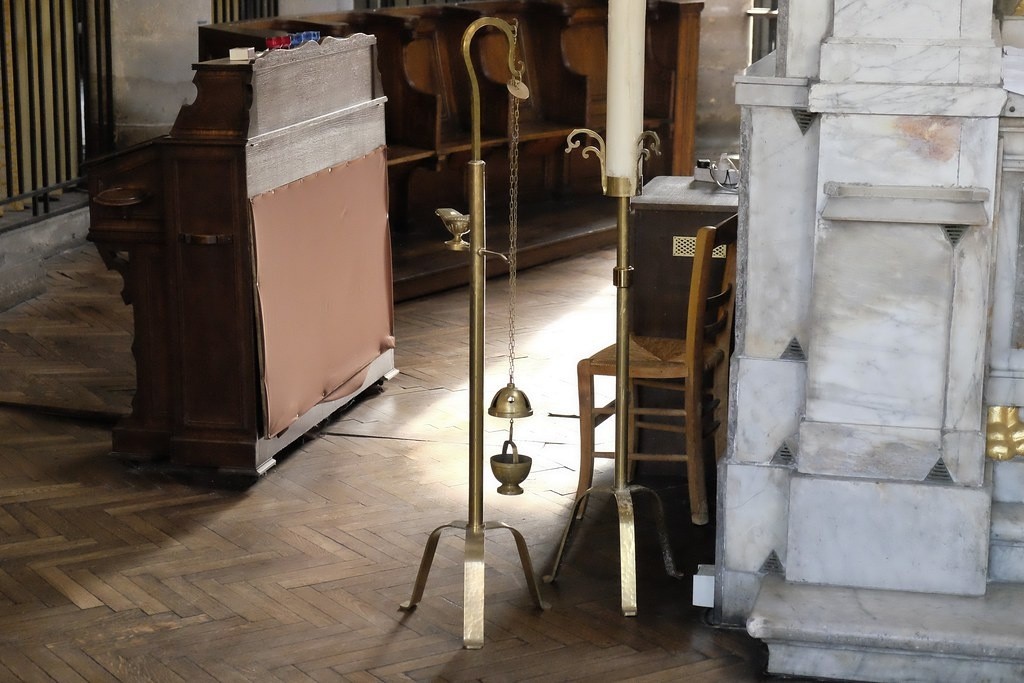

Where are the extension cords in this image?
left=694, top=166, right=740, bottom=184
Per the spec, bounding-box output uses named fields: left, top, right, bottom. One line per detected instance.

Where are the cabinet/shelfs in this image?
left=630, top=175, right=738, bottom=479
left=198, top=0, right=706, bottom=305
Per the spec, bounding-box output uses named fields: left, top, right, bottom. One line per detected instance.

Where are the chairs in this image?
left=576, top=213, right=739, bottom=526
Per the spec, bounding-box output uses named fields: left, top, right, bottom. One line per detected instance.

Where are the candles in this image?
left=605, top=0, right=646, bottom=177
